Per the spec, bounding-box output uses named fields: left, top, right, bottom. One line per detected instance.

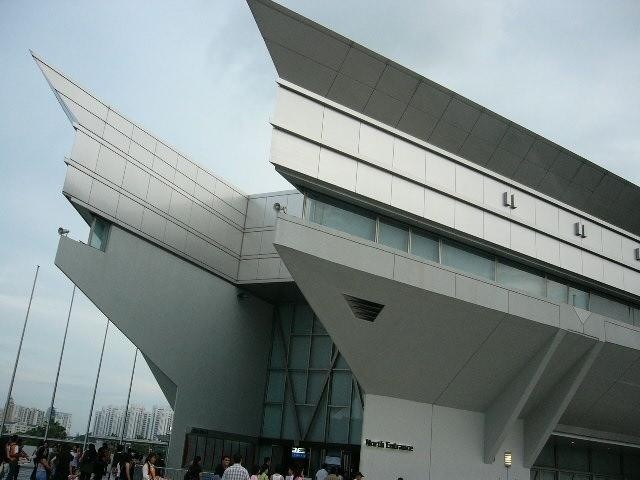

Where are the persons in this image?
left=214, top=453, right=365, bottom=480
left=0, top=435, right=168, bottom=480
left=183, top=455, right=204, bottom=480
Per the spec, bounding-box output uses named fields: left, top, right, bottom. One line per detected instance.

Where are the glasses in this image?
left=225, top=459, right=230, bottom=462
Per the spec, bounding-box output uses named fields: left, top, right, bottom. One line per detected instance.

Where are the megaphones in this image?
left=273, top=202, right=287, bottom=214
left=57, top=227, right=69, bottom=236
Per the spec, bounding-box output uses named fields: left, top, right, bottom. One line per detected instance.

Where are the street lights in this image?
left=504, top=449, right=512, bottom=480
